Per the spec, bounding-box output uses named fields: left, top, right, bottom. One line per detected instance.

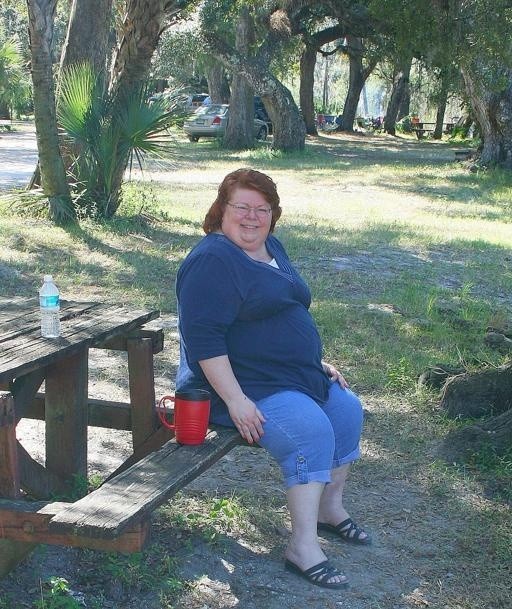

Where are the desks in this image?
left=0, top=304, right=166, bottom=584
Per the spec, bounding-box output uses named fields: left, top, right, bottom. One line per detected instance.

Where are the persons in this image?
left=174, top=168, right=374, bottom=589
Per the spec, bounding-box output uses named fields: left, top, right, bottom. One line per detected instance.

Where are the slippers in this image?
left=285, top=558, right=349, bottom=590
left=317, top=517, right=370, bottom=545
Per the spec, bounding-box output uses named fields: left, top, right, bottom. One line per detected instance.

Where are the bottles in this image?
left=40, top=274, right=60, bottom=338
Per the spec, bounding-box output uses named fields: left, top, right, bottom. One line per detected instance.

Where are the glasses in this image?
left=227, top=201, right=272, bottom=217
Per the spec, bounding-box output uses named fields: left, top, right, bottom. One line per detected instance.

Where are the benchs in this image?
left=48, top=387, right=243, bottom=547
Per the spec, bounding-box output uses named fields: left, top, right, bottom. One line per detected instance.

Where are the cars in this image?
left=175, top=93, right=274, bottom=141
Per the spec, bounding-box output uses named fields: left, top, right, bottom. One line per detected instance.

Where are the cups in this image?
left=158, top=388, right=212, bottom=446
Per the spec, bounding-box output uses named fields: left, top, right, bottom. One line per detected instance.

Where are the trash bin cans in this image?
left=357, top=118, right=365, bottom=127
